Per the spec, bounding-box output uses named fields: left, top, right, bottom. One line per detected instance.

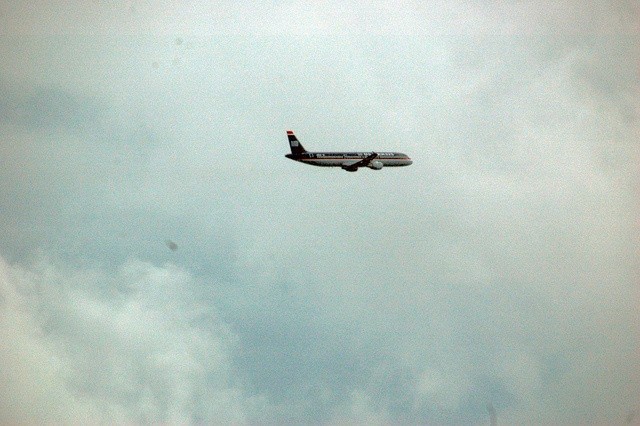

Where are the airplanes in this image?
left=284, top=130, right=413, bottom=171
